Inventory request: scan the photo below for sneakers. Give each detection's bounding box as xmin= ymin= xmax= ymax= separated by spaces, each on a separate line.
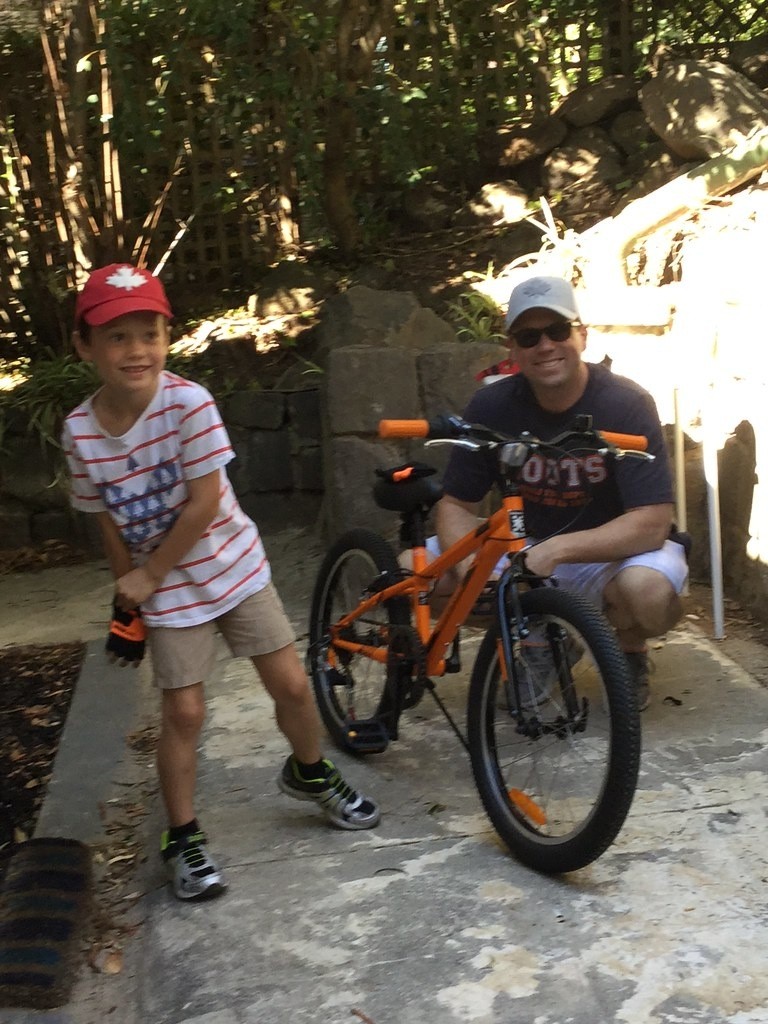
xmin=516 ymin=626 xmax=584 ymax=707
xmin=161 ymin=831 xmax=230 ymax=902
xmin=622 ymin=651 xmax=651 ymax=709
xmin=277 ymin=753 xmax=380 ymax=829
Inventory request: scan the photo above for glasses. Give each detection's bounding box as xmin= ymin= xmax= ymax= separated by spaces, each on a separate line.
xmin=508 ymin=322 xmax=575 ymax=348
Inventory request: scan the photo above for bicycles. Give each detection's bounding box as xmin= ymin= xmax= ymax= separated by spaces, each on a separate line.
xmin=301 ymin=411 xmax=659 ymax=876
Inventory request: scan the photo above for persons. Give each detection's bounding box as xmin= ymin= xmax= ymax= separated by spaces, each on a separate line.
xmin=63 ymin=263 xmax=380 ymax=902
xmin=396 ymin=277 xmax=692 ymax=712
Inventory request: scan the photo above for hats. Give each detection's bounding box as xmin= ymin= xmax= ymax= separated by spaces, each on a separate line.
xmin=508 ymin=277 xmax=577 ymax=330
xmin=75 ymin=264 xmax=174 ymax=325
